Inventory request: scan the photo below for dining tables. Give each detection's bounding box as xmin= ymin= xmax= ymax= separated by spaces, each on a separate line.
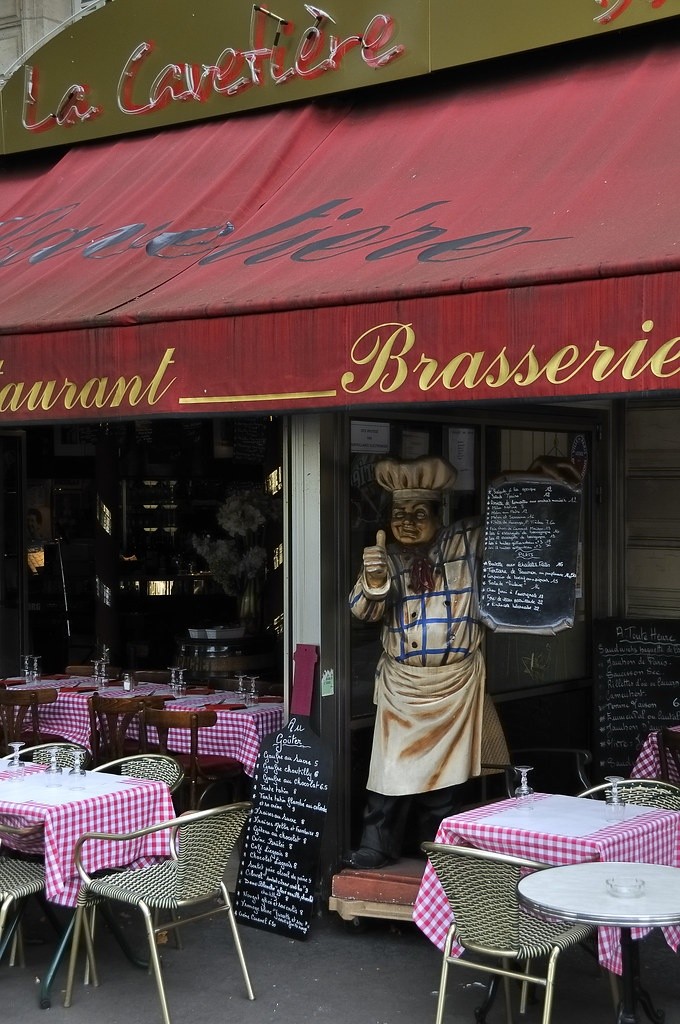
xmin=411 ymin=791 xmax=680 ymax=1024
xmin=0 ymin=674 xmax=284 ymax=810
xmin=0 ymin=760 xmax=177 ymax=1008
xmin=631 ymin=726 xmax=680 ymax=780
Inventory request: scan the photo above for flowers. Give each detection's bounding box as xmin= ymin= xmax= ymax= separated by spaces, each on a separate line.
xmin=192 ymin=482 xmax=277 ymax=598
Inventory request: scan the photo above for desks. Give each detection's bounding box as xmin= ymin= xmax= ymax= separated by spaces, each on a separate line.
xmin=514 ymin=861 xmax=680 ymax=1024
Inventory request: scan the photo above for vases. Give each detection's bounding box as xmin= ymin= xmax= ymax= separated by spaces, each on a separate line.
xmin=240 ymin=577 xmax=264 ymax=640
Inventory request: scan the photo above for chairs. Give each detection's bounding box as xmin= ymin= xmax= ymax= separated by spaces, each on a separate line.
xmin=138 ymin=708 xmax=242 ymax=812
xmin=0 ymin=838 xmax=95 ymax=985
xmin=91 ymin=753 xmax=183 ymax=973
xmin=64 ymin=801 xmax=255 ymax=1024
xmin=422 ymin=840 xmax=620 ymax=1024
xmin=7 ymin=742 xmax=90 ymax=770
xmin=0 ymin=689 xmax=58 ymax=761
xmin=87 ymin=692 xmax=166 ymax=775
xmin=575 ymin=780 xmax=680 ymax=812
xmin=503 ymin=746 xmax=592 ymax=798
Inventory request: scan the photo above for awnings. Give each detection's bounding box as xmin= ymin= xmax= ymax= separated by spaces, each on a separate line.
xmin=0 ymin=27 xmax=680 ymax=421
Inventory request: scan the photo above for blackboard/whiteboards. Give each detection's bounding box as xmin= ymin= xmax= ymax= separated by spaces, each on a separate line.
xmin=595 ymin=616 xmax=680 ymax=776
xmin=233 ymin=715 xmax=334 ymax=942
xmin=475 ymin=473 xmax=582 ymax=635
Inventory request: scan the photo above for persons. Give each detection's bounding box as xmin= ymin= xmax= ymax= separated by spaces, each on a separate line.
xmin=345 ymin=456 xmax=520 ymax=870
xmin=24 ymin=508 xmax=50 ymax=542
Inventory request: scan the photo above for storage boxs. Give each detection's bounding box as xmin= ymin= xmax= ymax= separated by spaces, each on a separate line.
xmin=206 ymin=627 xmax=245 ymax=639
xmin=188 ymin=628 xmax=206 ymax=639
